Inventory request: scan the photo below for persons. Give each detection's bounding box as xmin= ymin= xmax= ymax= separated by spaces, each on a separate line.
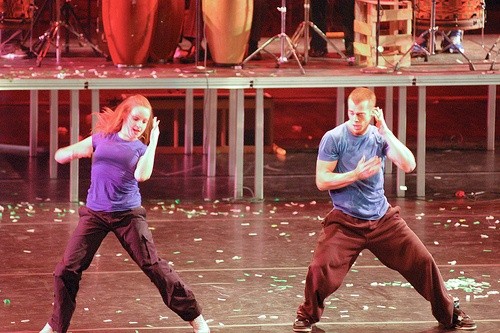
xmin=40 ymin=94 xmax=210 ymax=333
xmin=293 ymin=88 xmax=477 ymax=331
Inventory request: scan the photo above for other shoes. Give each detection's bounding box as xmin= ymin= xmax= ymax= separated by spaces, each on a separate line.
xmin=39 ymin=322 xmax=55 ymax=333
xmin=452 ymin=306 xmax=477 ymax=331
xmin=345 ymin=42 xmax=355 ymax=55
xmin=412 ymin=37 xmax=437 ymax=53
xmin=189 ymin=317 xmax=211 ymax=333
xmin=447 ymin=30 xmax=465 ymax=53
xmin=250 ymin=51 xmax=262 ymax=60
xmin=310 ymin=47 xmax=326 ymax=57
xmin=293 ymin=317 xmax=318 ymax=332
xmin=173 ymin=45 xmax=195 ymax=59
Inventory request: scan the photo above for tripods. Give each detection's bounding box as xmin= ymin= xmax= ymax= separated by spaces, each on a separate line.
xmin=392 ymin=0 xmax=500 ymax=73
xmin=237 ymin=0 xmax=353 ymax=74
xmin=0 ymin=0 xmax=110 ymax=67
